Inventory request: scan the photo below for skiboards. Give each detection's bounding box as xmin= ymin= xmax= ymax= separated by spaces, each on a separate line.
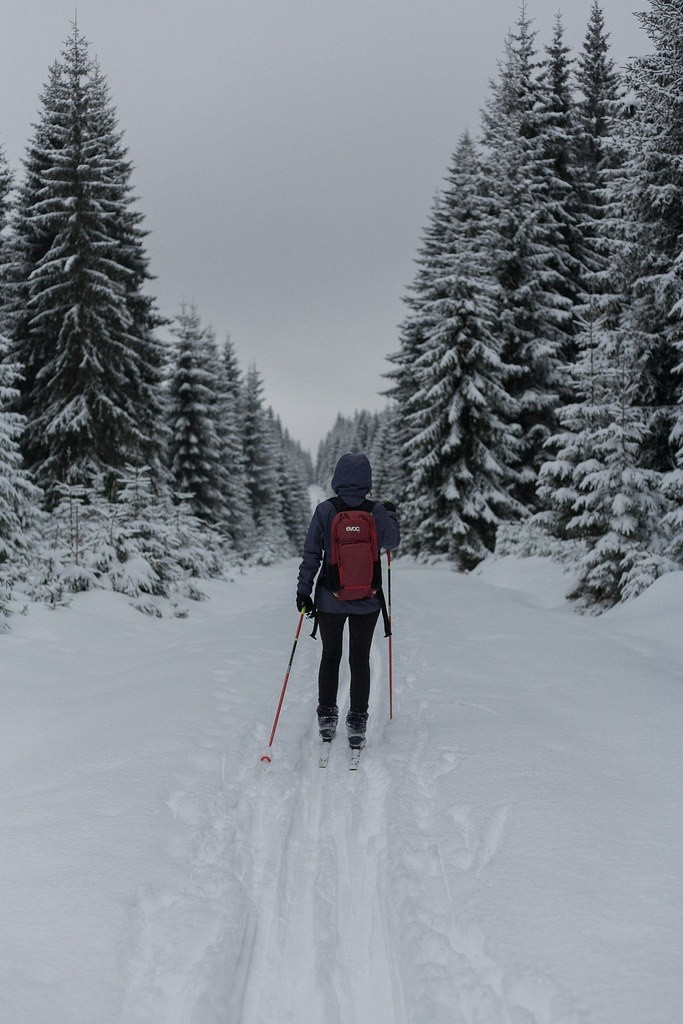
xmin=318 ymin=738 xmax=361 ymax=771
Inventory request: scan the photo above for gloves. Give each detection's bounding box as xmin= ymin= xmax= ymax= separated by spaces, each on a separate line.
xmin=384 ymin=501 xmax=396 ymax=511
xmin=296 ymin=591 xmax=313 ymax=613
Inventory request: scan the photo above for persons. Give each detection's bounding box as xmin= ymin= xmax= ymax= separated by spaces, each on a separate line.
xmin=296 ymin=453 xmax=402 ymax=749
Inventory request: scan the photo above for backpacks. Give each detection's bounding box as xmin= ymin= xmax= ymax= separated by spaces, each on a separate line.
xmin=320 ymin=496 xmax=383 ymax=600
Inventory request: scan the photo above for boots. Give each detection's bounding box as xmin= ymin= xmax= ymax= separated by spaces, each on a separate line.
xmin=346 ymin=711 xmax=368 ymax=744
xmin=316 ymin=705 xmax=338 ymax=739
